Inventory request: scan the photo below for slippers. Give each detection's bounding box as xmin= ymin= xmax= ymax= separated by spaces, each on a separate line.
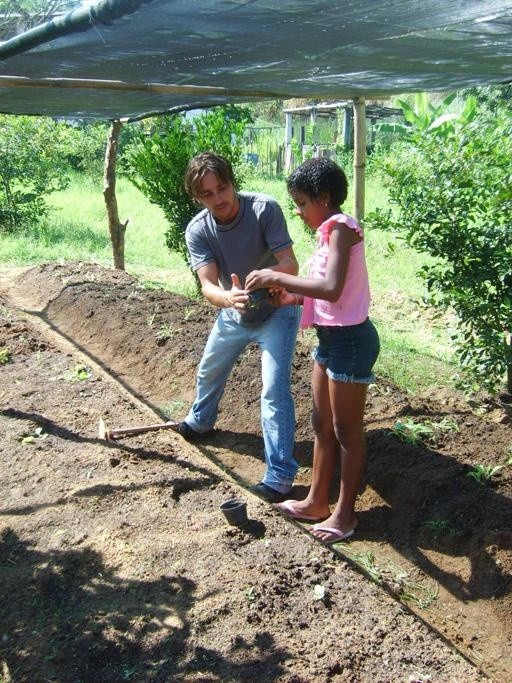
xmin=273 ymin=499 xmax=323 ymax=522
xmin=306 ymin=523 xmax=356 ymax=543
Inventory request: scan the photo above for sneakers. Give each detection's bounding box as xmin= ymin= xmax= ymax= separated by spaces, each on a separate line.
xmin=174 ymin=422 xmax=215 ymax=440
xmin=251 ymin=483 xmax=288 ymax=503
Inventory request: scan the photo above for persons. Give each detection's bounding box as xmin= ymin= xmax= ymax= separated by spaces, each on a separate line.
xmin=241 ymin=155 xmax=383 ymax=549
xmin=179 ymin=150 xmax=299 ymax=498
